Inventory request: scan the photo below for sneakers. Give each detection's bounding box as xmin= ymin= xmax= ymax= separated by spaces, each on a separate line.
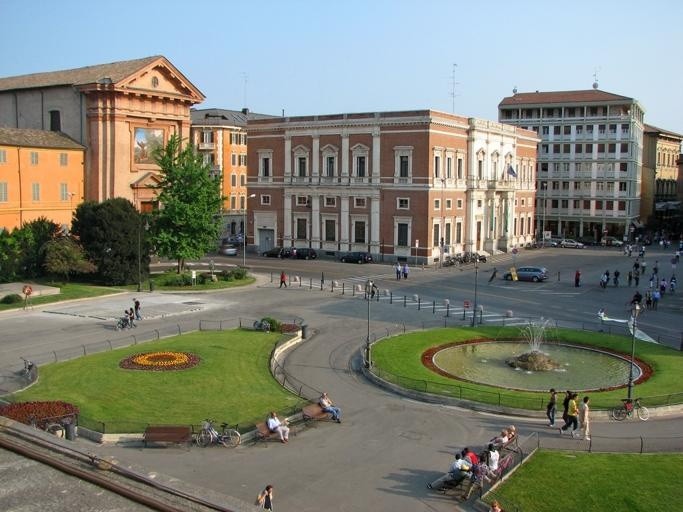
xmin=546 ymin=421 xmax=592 ymax=442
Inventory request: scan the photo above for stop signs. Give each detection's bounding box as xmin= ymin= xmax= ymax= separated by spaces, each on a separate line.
xmin=603 ymin=228 xmax=607 ymax=235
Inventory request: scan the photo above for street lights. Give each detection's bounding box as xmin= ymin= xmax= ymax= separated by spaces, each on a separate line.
xmin=241 ymin=193 xmax=256 ymax=266
xmin=363 ymin=279 xmax=374 ymax=369
xmin=625 ymin=300 xmax=639 ymax=398
xmin=542 ymin=182 xmax=547 ymax=249
xmin=68 ymin=191 xmax=75 ymax=221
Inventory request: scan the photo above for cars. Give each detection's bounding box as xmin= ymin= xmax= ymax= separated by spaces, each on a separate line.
xmin=538 ymin=238 xmax=558 ymax=249
xmin=219 ymin=233 xmax=248 ymax=257
xmin=561 ymin=239 xmax=584 ymax=249
xmin=339 ymin=250 xmax=373 ymax=265
xmin=503 ymin=265 xmax=549 ymax=282
xmin=262 ymin=247 xmax=317 ymax=260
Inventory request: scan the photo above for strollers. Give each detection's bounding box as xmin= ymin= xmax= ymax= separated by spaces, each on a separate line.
xmin=113 ymin=317 xmax=130 ymax=330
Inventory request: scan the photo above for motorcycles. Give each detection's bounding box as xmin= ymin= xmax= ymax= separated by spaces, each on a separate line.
xmin=18 ymin=357 xmax=34 ymax=389
xmin=443 ymin=250 xmax=488 ymax=267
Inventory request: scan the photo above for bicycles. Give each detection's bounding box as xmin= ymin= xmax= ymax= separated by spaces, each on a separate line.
xmin=611 ymin=397 xmax=650 ymax=422
xmin=196 ymin=416 xmax=243 ymax=449
xmin=669 ymin=279 xmax=678 ymax=295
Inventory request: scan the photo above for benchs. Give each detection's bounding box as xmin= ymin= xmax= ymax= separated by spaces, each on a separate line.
xmin=437 ymin=433 xmax=522 ymax=500
xmin=253 ymin=415 xmax=292 ymax=447
xmin=300 ymin=401 xmax=333 ymax=428
xmin=141 ymin=422 xmax=193 ymax=448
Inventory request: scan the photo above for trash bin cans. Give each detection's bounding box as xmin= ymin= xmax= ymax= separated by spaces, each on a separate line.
xmin=49 ymin=418 xmax=75 ymax=441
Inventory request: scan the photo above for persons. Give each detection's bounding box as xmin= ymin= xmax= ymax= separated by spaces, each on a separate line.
xmin=369 ymin=280 xmax=376 ymax=298
xmin=132 ymin=297 xmax=141 ymax=320
xmin=401 ymin=263 xmax=410 ymax=279
xmin=265 ymin=410 xmax=289 ymax=443
xmin=123 ymin=309 xmax=130 ymax=318
xmin=277 ymin=270 xmax=286 ymax=289
xmin=395 ymin=261 xmax=401 ymax=280
xmin=254 ymin=484 xmax=274 ymax=512
xmin=559 ymin=394 xmax=578 ymax=434
xmin=127 ymin=307 xmax=135 ymax=330
xmin=569 ymin=396 xmax=590 ymax=440
xmin=567 ymin=226 xmax=682 ymax=318
xmin=291 ymin=248 xmax=297 ymax=257
xmin=319 ymin=387 xmax=341 ymax=423
xmin=545 ymin=388 xmax=557 ymax=427
xmin=423 ymin=423 xmax=516 ymax=501
xmin=562 ymin=390 xmax=570 ymax=423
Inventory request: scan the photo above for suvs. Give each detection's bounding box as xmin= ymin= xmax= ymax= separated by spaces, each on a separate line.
xmin=600 ymin=236 xmax=624 ymax=247
